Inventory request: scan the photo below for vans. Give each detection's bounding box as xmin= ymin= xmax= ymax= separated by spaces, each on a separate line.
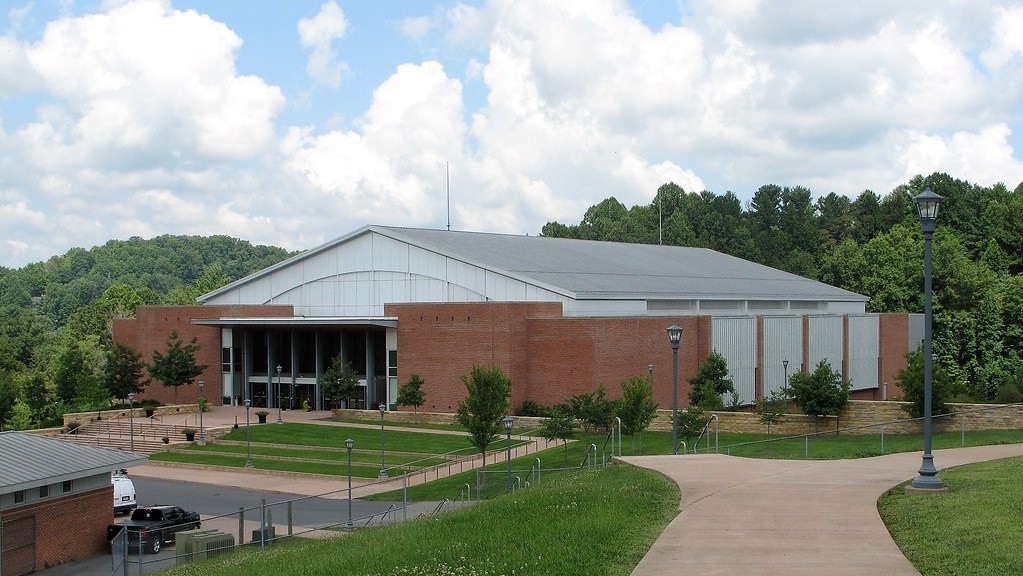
xmin=112 ymin=475 xmax=137 ymax=515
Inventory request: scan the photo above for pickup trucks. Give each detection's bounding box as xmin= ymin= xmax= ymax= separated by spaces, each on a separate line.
xmin=105 ymin=504 xmax=201 ymax=554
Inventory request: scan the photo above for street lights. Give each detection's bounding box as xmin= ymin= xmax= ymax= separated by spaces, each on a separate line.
xmin=276 ymin=365 xmax=282 ymax=421
xmin=648 ymin=363 xmax=654 ymax=410
xmin=198 ymin=381 xmax=206 ymax=440
xmin=783 ymin=358 xmax=788 ymax=408
xmin=129 ymin=392 xmax=135 ymax=452
xmin=378 ymin=402 xmax=388 ymax=475
xmin=244 ymin=398 xmax=253 ymax=466
xmin=666 ymin=321 xmax=683 ymax=453
xmin=914 ymin=185 xmax=942 ymax=490
xmin=932 ymin=354 xmax=937 ymax=383
xmin=502 ymin=415 xmax=514 ymax=493
xmin=345 ymin=438 xmax=354 ymax=529
